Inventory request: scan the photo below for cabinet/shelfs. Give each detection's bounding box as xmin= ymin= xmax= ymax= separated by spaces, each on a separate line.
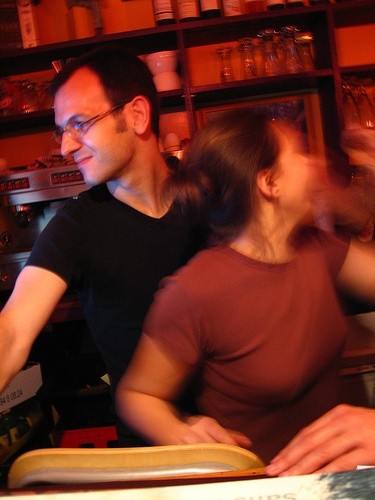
xmin=0 ymin=0 xmax=375 ymax=376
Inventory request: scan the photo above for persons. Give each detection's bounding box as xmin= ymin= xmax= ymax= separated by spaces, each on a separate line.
xmin=114 ymin=105 xmax=375 ymax=478
xmin=0 ymin=50 xmax=222 ymax=449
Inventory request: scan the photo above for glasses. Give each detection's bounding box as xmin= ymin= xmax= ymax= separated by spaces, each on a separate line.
xmin=50 ymin=102 xmax=130 ymax=143
